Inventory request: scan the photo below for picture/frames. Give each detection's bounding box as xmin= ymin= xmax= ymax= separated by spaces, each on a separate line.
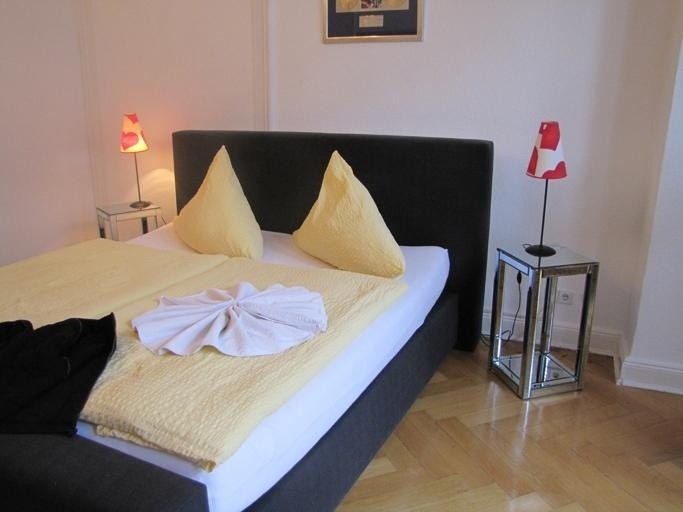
xmin=320 ymin=0 xmax=424 ymax=43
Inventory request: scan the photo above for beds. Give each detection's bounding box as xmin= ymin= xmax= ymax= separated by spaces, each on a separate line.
xmin=0 ymin=129 xmax=494 ymax=512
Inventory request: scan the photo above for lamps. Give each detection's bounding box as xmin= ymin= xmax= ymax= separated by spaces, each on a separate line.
xmin=526 ymin=120 xmax=568 ymax=257
xmin=120 ymin=112 xmax=150 ymax=208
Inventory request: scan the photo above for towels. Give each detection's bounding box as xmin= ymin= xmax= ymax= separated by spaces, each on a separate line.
xmin=131 ymin=281 xmax=329 ymax=358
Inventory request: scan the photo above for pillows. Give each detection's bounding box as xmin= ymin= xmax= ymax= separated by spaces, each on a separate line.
xmin=172 ymin=144 xmax=407 ymax=278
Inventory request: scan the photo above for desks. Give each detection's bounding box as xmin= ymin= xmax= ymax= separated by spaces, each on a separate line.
xmin=489 ymin=244 xmax=602 ymax=401
xmin=95 ymin=202 xmax=161 ymax=241
